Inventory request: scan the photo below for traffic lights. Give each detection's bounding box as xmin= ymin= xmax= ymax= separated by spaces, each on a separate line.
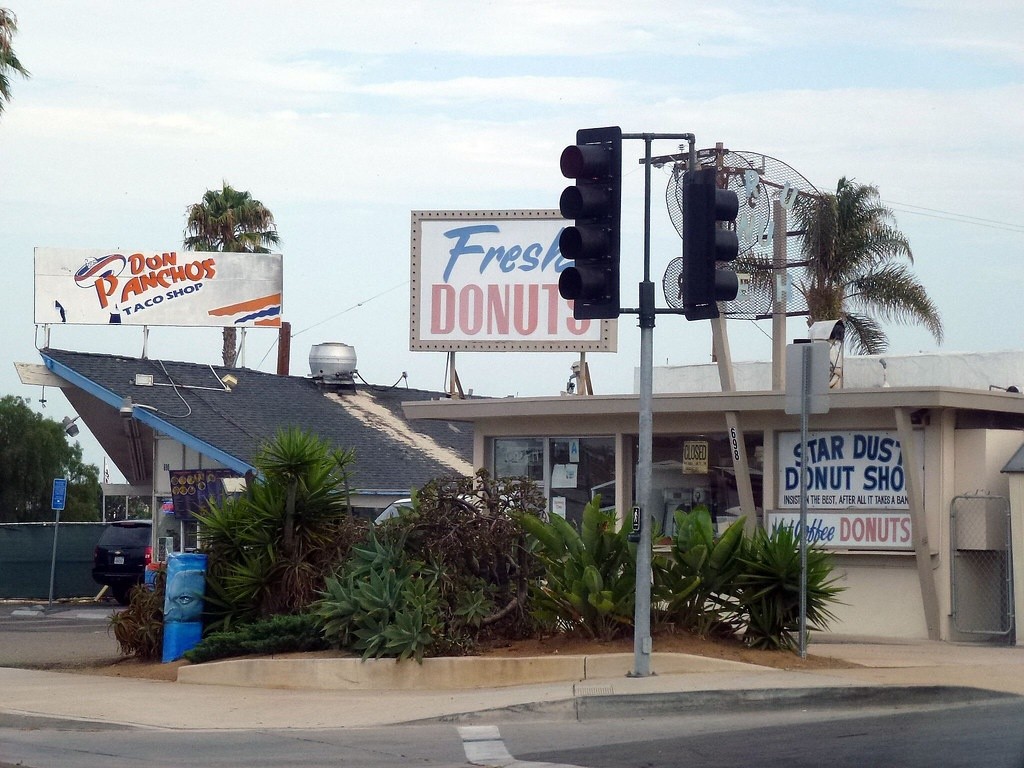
xmin=560 ymin=127 xmax=621 ymax=320
xmin=682 ymin=167 xmax=740 ymax=320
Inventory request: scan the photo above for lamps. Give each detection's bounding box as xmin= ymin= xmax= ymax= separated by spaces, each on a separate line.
xmin=221 ymin=374 xmax=238 ymax=393
xmin=62 ymin=415 xmax=81 ymax=437
xmin=135 ymin=374 xmax=153 ymax=387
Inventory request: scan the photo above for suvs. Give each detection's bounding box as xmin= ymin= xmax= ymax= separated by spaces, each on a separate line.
xmin=374 ymin=499 xmax=508 ymax=522
xmin=91 ymin=500 xmax=176 ymax=607
